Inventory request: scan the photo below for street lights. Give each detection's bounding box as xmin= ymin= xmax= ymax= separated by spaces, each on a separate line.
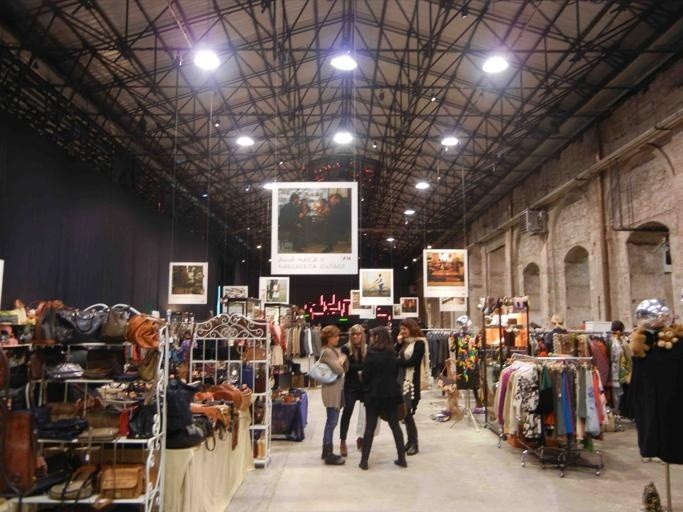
xmin=27 ymin=405 xmax=162 ymax=441
xmin=307 ymin=350 xmax=338 ymax=384
xmin=166 ymin=366 xmax=195 ymax=432
xmin=102 ymin=468 xmax=142 ymax=499
xmin=125 ymin=314 xmax=164 ymax=348
xmin=381 ymin=399 xmax=408 ymax=421
xmin=50 ymin=471 xmax=93 ymax=504
xmin=101 ymin=310 xmax=127 ymax=342
xmin=32 ymin=300 xmax=63 ymax=342
xmin=26 ymin=348 xmax=159 ymax=382
xmin=55 ymin=307 xmax=106 ymax=342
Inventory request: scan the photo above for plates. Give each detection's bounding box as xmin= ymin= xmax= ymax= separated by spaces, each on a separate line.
xmin=0 ymin=304 xmax=168 ymax=512
xmin=481 ymin=296 xmax=529 ymax=427
xmin=187 ymin=313 xmax=274 ymax=469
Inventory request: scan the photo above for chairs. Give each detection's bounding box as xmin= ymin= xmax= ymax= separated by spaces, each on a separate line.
xmin=395 ymin=459 xmax=407 ymax=467
xmin=359 ymin=462 xmax=367 ymax=470
xmin=407 ymin=445 xmax=417 ymax=455
xmin=405 ymin=442 xmax=409 ymax=451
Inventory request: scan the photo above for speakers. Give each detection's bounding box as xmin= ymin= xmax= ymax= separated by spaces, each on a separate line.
xmin=324 ymin=444 xmax=345 ymax=465
xmin=340 ymin=440 xmax=346 ymax=456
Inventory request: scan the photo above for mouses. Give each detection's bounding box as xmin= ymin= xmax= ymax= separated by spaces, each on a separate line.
xmin=542 ymin=327 xmax=629 ymax=340
xmin=425 ymin=326 xmax=455 ymax=335
xmin=646 ymin=310 xmax=680 ymax=330
xmin=507 ymin=355 xmax=599 ymax=384
xmin=509 ymin=352 xmax=542 ymax=364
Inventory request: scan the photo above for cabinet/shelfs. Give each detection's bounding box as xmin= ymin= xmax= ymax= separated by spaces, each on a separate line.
xmin=481 ymin=296 xmax=529 ymax=427
xmin=0 ymin=304 xmax=168 ymax=512
xmin=187 ymin=313 xmax=274 ymax=469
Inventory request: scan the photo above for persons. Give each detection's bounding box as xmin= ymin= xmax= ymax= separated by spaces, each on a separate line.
xmin=377 ymin=275 xmax=384 ymax=292
xmin=544 ymin=313 xmax=567 ymax=353
xmin=339 ymin=325 xmax=371 ymax=456
xmin=359 ymin=328 xmax=408 ymax=470
xmin=278 ymin=194 xmax=346 ymax=251
xmin=172 ymin=330 xmax=198 ymax=364
xmin=317 ymin=325 xmax=344 ymax=465
xmin=271 ymin=280 xmax=280 ymax=302
xmin=393 ymin=318 xmax=424 ymax=454
xmin=613 ymin=320 xmax=624 ymax=330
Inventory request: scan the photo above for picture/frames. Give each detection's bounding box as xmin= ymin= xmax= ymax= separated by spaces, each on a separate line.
xmin=164 ymin=403 xmax=255 ymax=512
xmin=272 ymin=388 xmax=308 ymax=442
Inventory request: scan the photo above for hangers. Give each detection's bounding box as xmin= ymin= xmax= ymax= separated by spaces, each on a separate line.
xmin=425 ymin=326 xmax=455 ymax=335
xmin=509 ymin=352 xmax=542 ymax=364
xmin=542 ymin=327 xmax=629 ymax=340
xmin=646 ymin=310 xmax=680 ymax=330
xmin=507 ymin=355 xmax=599 ymax=384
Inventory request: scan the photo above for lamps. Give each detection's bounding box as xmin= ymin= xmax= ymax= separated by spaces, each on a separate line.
xmin=191 ymin=42 xmax=512 ymax=271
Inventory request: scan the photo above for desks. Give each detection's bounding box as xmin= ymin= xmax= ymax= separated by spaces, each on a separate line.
xmin=164 ymin=403 xmax=255 ymax=512
xmin=272 ymin=388 xmax=308 ymax=442
